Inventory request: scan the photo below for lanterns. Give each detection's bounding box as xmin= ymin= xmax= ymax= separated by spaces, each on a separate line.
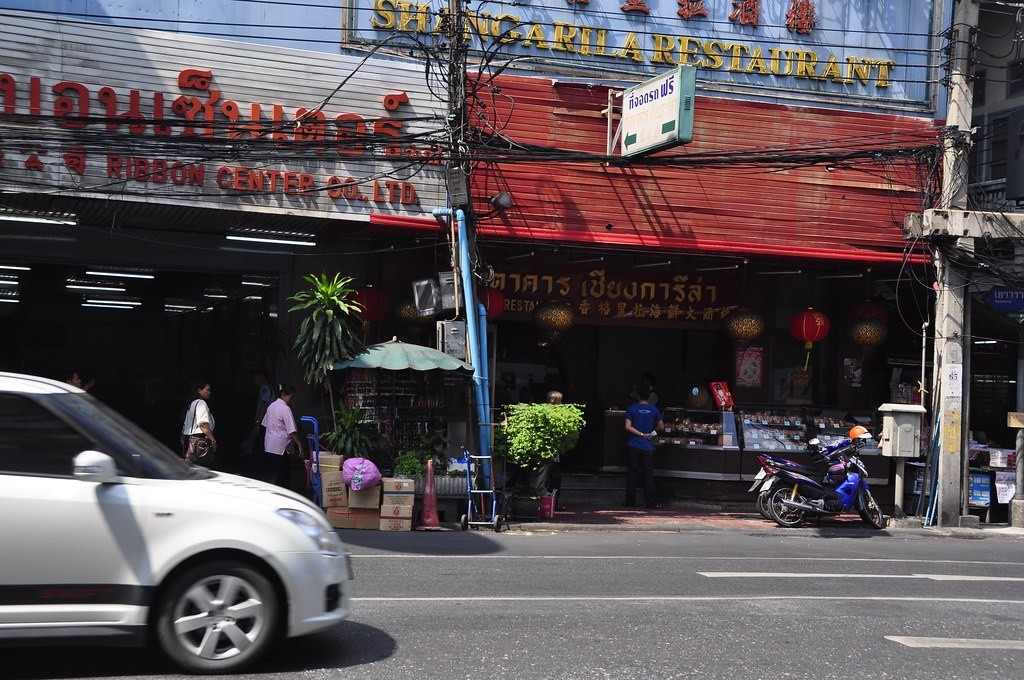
xmin=789 ymin=306 xmax=830 ymax=351
xmin=847 ymin=315 xmax=889 ymax=351
xmin=724 ymin=310 xmax=765 ymax=343
xmin=348 ymin=286 xmax=388 ymax=324
xmin=478 ymin=287 xmax=506 ymax=321
xmin=532 ymin=299 xmax=575 ymax=336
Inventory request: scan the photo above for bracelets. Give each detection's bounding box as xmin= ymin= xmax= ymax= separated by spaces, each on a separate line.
xmin=651 ymin=430 xmax=658 ymax=437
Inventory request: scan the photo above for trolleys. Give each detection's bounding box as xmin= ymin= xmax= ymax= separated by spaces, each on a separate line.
xmin=300 ymin=415 xmax=324 ymax=509
xmin=460 ymin=445 xmax=510 ymax=533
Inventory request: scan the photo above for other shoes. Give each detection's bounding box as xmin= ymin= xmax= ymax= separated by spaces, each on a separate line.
xmin=647 ymin=503 xmax=665 ymax=509
xmin=555 ymin=504 xmax=566 ymax=511
xmin=620 ymin=501 xmax=640 ymax=507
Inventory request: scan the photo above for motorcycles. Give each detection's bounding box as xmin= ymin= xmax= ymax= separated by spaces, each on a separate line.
xmin=747 ymin=432 xmax=883 ymax=530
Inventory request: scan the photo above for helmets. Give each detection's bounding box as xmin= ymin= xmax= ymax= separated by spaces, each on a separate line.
xmin=850 ymin=426 xmax=872 ymax=440
xmin=827 ymin=463 xmax=847 ymax=483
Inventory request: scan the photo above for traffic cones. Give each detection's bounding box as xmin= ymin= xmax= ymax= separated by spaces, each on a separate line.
xmin=415 ymin=460 xmax=453 ymax=532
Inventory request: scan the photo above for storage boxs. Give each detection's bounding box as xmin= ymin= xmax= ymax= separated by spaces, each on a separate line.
xmin=541 ymin=494 xmax=556 ymax=518
xmin=990 ymin=450 xmax=1016 ymax=467
xmin=312 ymin=450 xmax=416 ymax=532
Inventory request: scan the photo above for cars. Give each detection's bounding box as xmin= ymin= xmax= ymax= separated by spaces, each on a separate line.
xmin=0 ymin=372 xmax=355 ymax=673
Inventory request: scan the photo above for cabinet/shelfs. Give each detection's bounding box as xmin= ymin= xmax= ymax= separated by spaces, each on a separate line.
xmin=655 ymin=406 xmax=877 ymax=450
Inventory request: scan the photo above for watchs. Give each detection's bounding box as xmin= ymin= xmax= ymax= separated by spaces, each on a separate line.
xmin=211 ymin=440 xmax=216 ymax=444
xmin=641 ymin=432 xmax=644 ymax=437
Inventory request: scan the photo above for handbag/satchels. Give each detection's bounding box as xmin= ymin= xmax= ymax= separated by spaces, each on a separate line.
xmin=540 ymin=496 xmax=554 ymax=518
xmin=186 ymin=437 xmax=215 ymax=463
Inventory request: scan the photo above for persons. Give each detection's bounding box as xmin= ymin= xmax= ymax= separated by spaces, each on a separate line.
xmin=62 ymin=370 xmax=100 ymax=400
xmin=618 ymin=384 xmax=665 ymax=507
xmin=181 ymin=379 xmax=219 ymax=460
xmin=261 ymin=385 xmax=306 ymax=474
xmin=542 ymin=391 xmax=566 ymax=511
xmin=249 ymin=364 xmax=276 ymax=462
xmin=639 ymin=372 xmax=664 ymax=407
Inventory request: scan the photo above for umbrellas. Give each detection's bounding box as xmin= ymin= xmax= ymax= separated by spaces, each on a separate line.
xmin=331 ymin=335 xmax=475 ymax=472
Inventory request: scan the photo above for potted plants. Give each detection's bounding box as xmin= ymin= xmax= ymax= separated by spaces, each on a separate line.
xmin=392 ymin=436 xmax=476 ymax=496
xmin=492 ymin=402 xmax=587 ymax=519
